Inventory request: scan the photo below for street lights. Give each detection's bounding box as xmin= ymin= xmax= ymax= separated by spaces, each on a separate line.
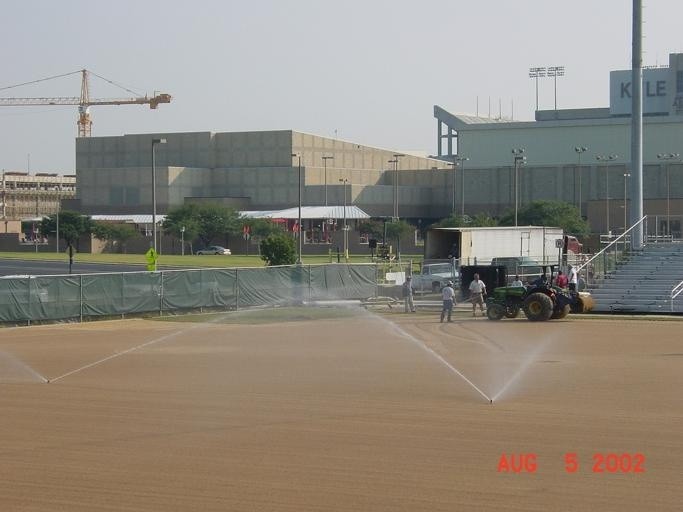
xmin=548 ymin=64 xmax=564 ymax=111
xmin=445 ymin=163 xmax=460 ymax=215
xmin=622 ymin=173 xmax=631 ymax=251
xmin=337 ymin=178 xmax=350 ymax=257
xmin=52 ymin=185 xmax=60 ymax=254
xmin=394 ymin=154 xmax=404 ymax=222
xmin=321 ymin=155 xmax=333 ymax=206
xmin=148 ymin=136 xmax=169 ymax=272
xmin=572 ymin=146 xmax=589 ymax=224
xmin=595 ymin=154 xmax=619 ymax=240
xmin=292 ymin=151 xmax=303 ymax=267
xmin=510 ymin=148 xmax=526 ymax=228
xmin=514 ymin=156 xmax=527 ymax=208
xmin=456 ymin=157 xmax=468 ymax=216
xmin=529 ymin=67 xmax=548 ymax=111
xmin=657 ymin=151 xmax=680 ymax=237
xmin=388 ymin=159 xmax=399 ymax=218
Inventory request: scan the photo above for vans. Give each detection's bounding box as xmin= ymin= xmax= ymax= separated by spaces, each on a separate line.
xmin=489 ymin=255 xmax=555 ymax=289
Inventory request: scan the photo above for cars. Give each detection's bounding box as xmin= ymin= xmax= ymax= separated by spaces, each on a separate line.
xmin=195 ymin=245 xmax=231 ymax=255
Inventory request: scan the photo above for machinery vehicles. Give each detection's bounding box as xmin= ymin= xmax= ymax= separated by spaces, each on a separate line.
xmin=480 ymin=261 xmax=598 ymax=323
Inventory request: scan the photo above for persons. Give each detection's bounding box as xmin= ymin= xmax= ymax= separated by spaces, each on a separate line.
xmin=511 ymin=274 xmax=523 ymax=287
xmin=555 ymin=270 xmax=568 ymax=288
xmin=440 ymin=280 xmax=457 ymax=323
xmin=468 ymin=272 xmax=488 ymax=317
xmin=566 ymin=263 xmax=578 ymax=313
xmin=401 ymin=276 xmax=416 ymax=313
xmin=529 ymin=275 xmax=546 ymax=286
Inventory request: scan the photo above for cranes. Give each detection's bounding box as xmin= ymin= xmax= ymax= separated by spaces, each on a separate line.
xmin=0 ymin=68 xmax=171 ymax=138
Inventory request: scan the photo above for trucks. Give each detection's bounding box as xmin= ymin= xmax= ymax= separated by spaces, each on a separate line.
xmin=399 ymin=261 xmax=459 ymax=294
xmin=419 ymin=221 xmax=588 ymax=281
